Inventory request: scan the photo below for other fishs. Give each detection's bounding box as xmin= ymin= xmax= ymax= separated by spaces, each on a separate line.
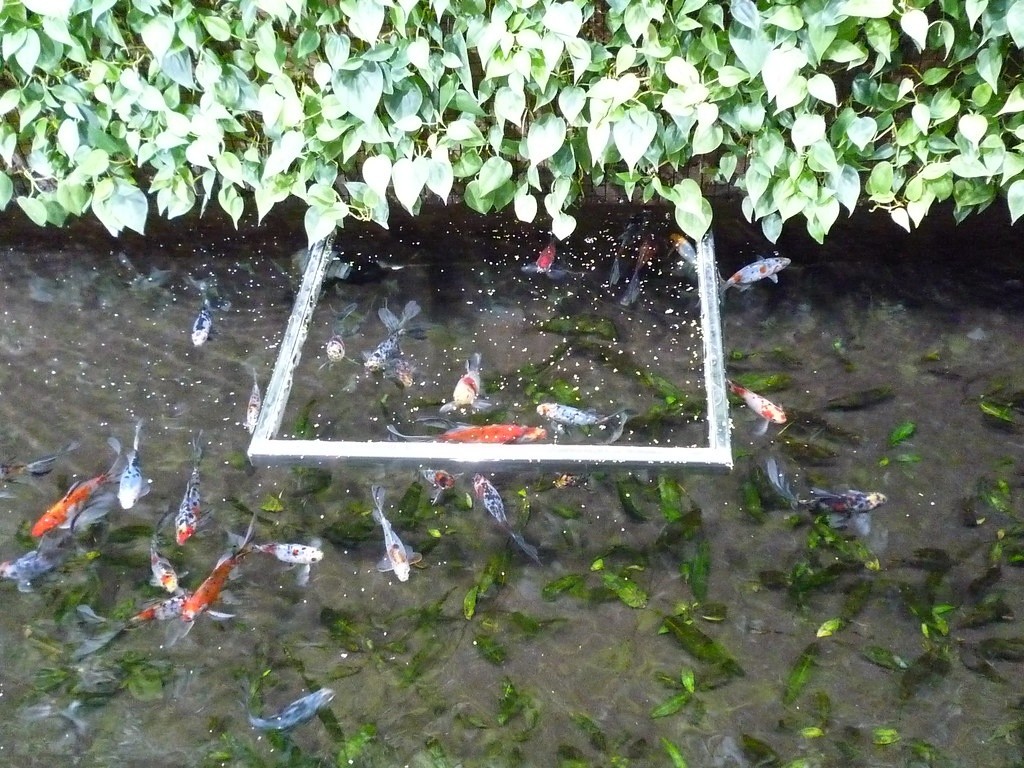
xmin=0 ymin=236 xmax=896 ymax=766
